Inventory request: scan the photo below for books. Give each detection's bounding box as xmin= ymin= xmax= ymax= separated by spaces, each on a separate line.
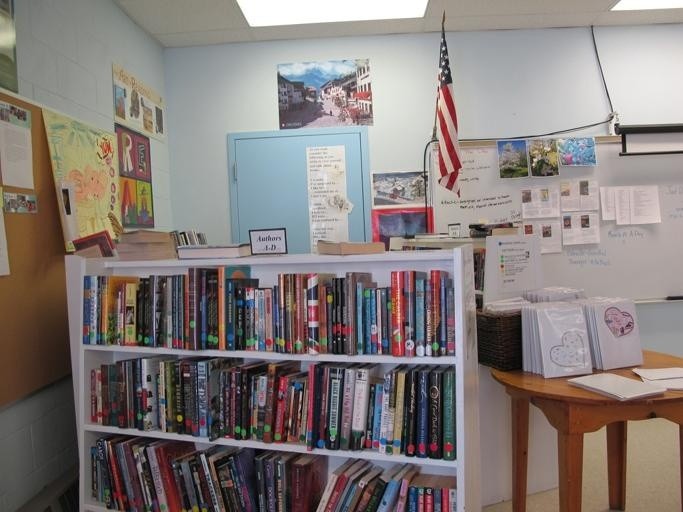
xmin=176 ymin=244 xmax=251 ymax=259
xmin=169 ymin=229 xmax=207 ymax=245
xmin=72 ymin=244 xmax=102 ymax=257
xmin=475 ymin=285 xmax=645 ymax=381
xmin=71 ymin=230 xmax=117 ymax=257
xmin=567 ymin=371 xmax=666 ymax=405
xmin=90 ymin=357 xmax=455 ymax=460
xmin=83 ymin=265 xmax=454 ymax=357
xmin=115 ymin=230 xmax=175 ymax=261
xmin=90 ymin=436 xmax=457 ymax=511
xmin=316 ymin=240 xmax=386 ymax=256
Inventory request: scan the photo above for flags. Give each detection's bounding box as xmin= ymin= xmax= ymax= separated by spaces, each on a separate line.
xmin=435 ymin=21 xmax=462 ymax=198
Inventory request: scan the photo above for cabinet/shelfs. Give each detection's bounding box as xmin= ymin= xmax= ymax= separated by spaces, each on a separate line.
xmin=65 ymin=244 xmax=483 ymax=512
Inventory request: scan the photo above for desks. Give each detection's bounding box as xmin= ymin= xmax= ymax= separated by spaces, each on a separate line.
xmin=490 ymin=349 xmax=683 ymax=512
